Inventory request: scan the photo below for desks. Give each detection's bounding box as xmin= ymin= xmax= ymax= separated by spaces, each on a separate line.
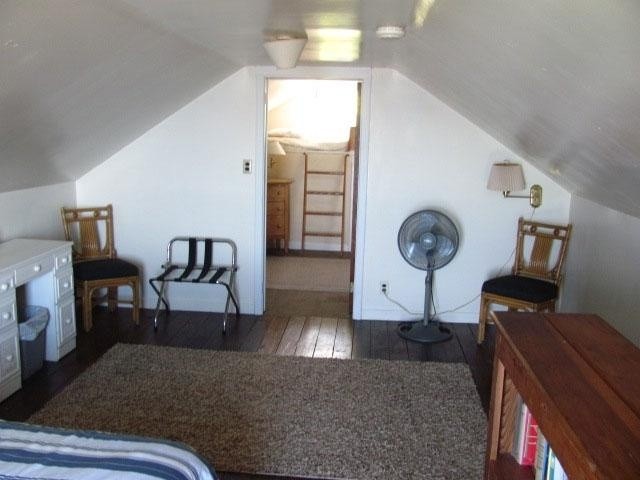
xmin=1 ymin=237 xmax=77 ymax=404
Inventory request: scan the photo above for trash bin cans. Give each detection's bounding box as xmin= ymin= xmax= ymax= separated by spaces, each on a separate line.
xmin=18 ymin=305 xmax=50 ymax=381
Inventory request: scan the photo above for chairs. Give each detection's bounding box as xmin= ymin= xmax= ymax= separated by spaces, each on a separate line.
xmin=61 ymin=204 xmax=141 ymax=332
xmin=149 ymin=237 xmax=240 ymax=335
xmin=477 ymin=217 xmax=572 ymax=343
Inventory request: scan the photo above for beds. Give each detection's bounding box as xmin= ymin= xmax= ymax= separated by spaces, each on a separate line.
xmin=0 ymin=417 xmax=219 ymax=479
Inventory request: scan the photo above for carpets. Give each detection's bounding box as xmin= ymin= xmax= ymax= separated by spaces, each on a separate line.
xmin=19 ymin=342 xmax=490 ymax=479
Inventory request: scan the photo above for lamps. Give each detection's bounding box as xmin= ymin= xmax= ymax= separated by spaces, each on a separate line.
xmin=485 ymin=160 xmax=542 ymax=207
xmin=261 ymin=33 xmax=307 ymax=71
xmin=267 ymin=141 xmax=286 ymax=179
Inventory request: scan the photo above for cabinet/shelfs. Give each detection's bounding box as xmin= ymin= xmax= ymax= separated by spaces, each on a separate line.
xmin=481 ymin=310 xmax=638 ymax=479
xmin=267 ymin=179 xmax=292 ymax=255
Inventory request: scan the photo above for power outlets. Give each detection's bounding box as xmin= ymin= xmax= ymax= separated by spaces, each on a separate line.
xmin=380 ymin=282 xmax=390 ymax=297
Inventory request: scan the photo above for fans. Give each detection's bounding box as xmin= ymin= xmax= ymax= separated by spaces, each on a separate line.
xmin=398 ymin=209 xmax=459 ymax=344
